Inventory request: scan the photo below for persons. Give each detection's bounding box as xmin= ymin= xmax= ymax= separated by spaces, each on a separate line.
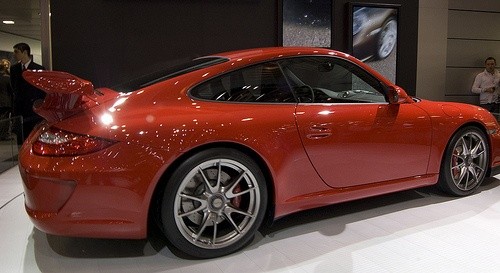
xmin=0 ymin=42 xmax=46 ymax=151
xmin=472 ymin=57 xmax=500 ymax=113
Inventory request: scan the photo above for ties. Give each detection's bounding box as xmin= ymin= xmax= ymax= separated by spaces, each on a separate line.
xmin=23 ymin=64 xmax=26 ymax=70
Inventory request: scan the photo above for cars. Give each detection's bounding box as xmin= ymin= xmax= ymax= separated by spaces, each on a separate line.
xmin=18 ymin=45 xmax=500 ymax=259
